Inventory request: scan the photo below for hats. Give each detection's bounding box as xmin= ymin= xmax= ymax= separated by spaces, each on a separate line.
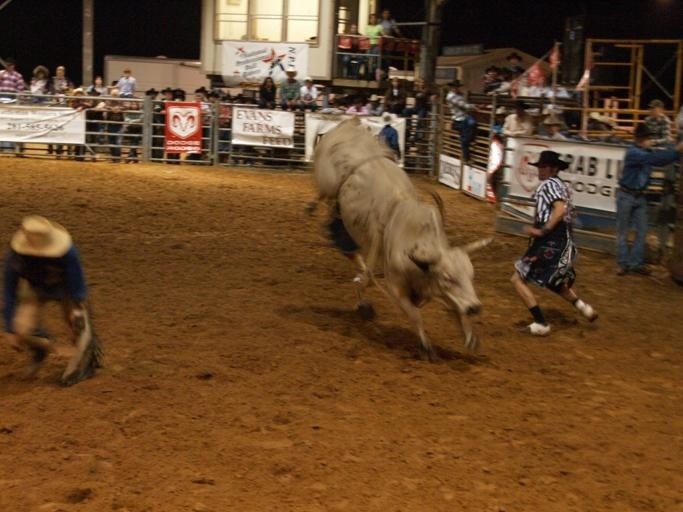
xmin=632 ymin=123 xmax=657 ymax=137
xmin=145 ymin=86 xmax=254 ymax=105
xmin=285 ymin=66 xmax=297 ymax=72
xmin=484 ymin=66 xmax=502 ymax=74
xmin=528 ymin=151 xmax=571 ymax=170
xmin=505 ymin=51 xmax=522 ymax=60
xmin=10 ymin=216 xmax=73 ymax=259
xmin=34 ymin=66 xmax=49 ymax=77
xmin=382 ymin=112 xmax=394 ymax=125
xmin=447 ymin=80 xmax=464 ymax=88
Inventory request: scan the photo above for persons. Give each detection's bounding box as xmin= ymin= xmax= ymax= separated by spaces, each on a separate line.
xmin=362 ymin=13 xmax=386 ymax=81
xmin=443 ymin=50 xmax=681 ymax=239
xmin=376 ymin=7 xmax=400 ymax=40
xmin=340 ymin=22 xmax=366 ymax=79
xmin=1 ymin=59 xmax=435 ymax=172
xmin=1 ymin=212 xmax=96 ymax=385
xmin=611 ymin=122 xmax=681 ymax=276
xmin=510 ymin=149 xmax=597 ymax=337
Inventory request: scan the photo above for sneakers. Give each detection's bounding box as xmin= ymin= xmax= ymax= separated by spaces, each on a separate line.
xmin=581 ymin=303 xmax=598 ymax=321
xmin=630 ymin=266 xmax=651 ymax=274
xmin=149 ymin=156 xmax=290 ymax=168
xmin=527 ymin=322 xmax=550 ymax=335
xmin=16 ymin=154 xmax=21 ymax=158
xmin=20 ymin=154 xmax=24 ymax=158
xmin=47 ymin=152 xmax=139 ymax=163
xmin=470 ymin=143 xmax=475 ymax=146
xmin=463 ymin=160 xmax=473 ymax=165
xmin=615 ymin=265 xmax=627 ymax=275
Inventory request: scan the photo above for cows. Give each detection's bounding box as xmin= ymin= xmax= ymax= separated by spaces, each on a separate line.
xmin=303 ymin=113 xmax=496 ymax=365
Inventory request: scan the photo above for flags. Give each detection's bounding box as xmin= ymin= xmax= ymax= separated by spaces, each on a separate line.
xmin=336 ymin=35 xmax=419 ymax=55
